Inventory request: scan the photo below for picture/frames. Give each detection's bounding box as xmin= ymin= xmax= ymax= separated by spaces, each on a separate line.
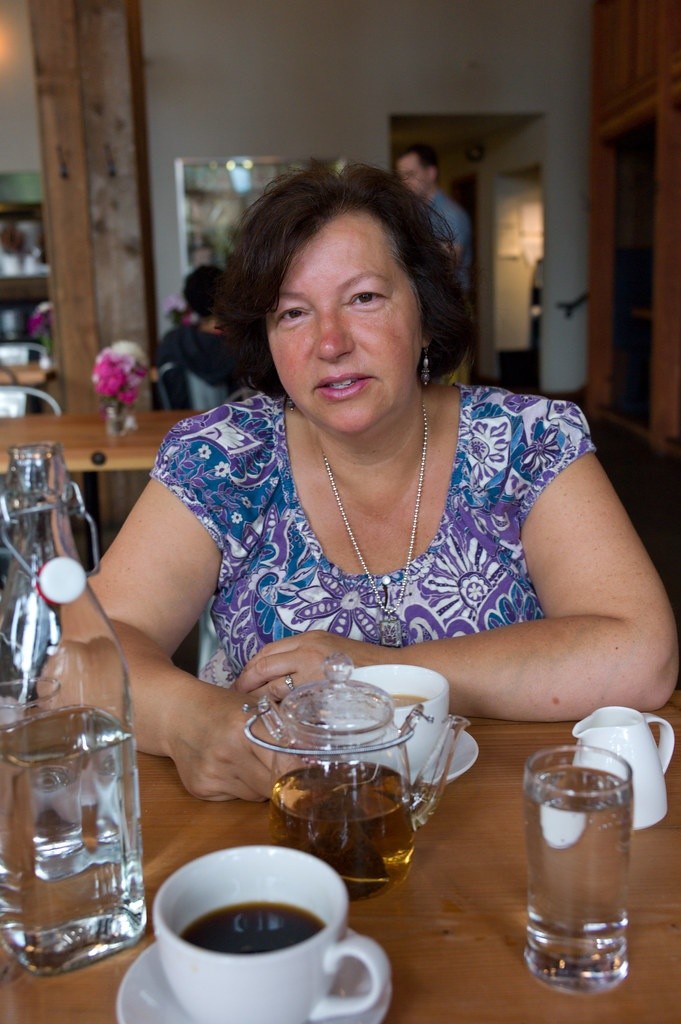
xmin=175 ymin=153 xmax=309 ymax=276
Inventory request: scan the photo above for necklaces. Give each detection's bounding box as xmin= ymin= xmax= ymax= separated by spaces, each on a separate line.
xmin=321 ymin=401 xmax=427 ymax=647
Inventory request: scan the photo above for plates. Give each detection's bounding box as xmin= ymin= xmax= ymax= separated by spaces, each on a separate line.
xmin=414 ymin=727 xmax=479 ymax=784
xmin=115 ymin=922 xmax=392 ymax=1024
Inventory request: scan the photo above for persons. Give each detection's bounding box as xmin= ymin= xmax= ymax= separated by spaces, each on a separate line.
xmin=155 ymin=265 xmax=238 ymax=410
xmin=396 ymin=143 xmax=469 ymax=384
xmin=38 ymin=157 xmax=679 ymax=802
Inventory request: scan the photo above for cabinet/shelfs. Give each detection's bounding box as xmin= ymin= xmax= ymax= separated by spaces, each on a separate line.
xmin=591 ymin=1 xmax=681 ymax=454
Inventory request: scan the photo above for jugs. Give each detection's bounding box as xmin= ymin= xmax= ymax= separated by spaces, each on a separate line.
xmin=243 ymin=653 xmax=470 ymax=900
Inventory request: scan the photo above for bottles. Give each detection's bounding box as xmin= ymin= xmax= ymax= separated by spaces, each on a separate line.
xmin=0 ymin=443 xmax=148 ymax=979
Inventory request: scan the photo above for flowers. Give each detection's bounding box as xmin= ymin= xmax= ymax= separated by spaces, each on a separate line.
xmin=30 ymin=299 xmax=57 ymax=359
xmin=93 ymin=339 xmax=149 ymax=414
xmin=165 ymin=292 xmax=192 ymax=327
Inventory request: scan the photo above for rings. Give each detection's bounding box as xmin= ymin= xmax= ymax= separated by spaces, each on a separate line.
xmin=286 ymin=675 xmax=294 ymax=691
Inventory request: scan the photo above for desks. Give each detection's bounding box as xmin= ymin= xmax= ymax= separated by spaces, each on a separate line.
xmin=1 ymin=687 xmax=680 ymax=1024
xmin=0 ymin=408 xmax=210 ymax=569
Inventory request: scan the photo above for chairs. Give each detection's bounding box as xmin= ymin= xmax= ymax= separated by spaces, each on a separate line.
xmin=0 ymin=385 xmax=100 ymax=587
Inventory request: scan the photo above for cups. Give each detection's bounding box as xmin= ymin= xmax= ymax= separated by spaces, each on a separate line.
xmin=348 ymin=665 xmax=449 ymax=773
xmin=570 ymin=705 xmax=676 ymax=829
xmin=153 ymin=846 xmax=388 ymax=1024
xmin=522 ymin=746 xmax=636 ymax=994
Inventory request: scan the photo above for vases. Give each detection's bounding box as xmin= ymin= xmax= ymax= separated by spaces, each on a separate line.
xmin=105 ymin=404 xmax=125 ymax=437
